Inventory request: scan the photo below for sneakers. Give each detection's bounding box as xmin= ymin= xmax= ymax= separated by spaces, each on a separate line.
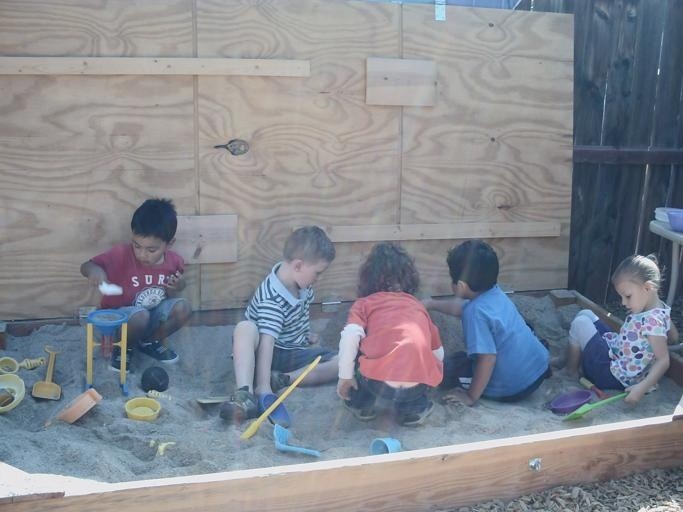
xmin=271 ymin=367 xmax=291 ymax=394
xmin=343 ymin=387 xmax=376 ymax=421
xmin=400 ymin=400 xmax=435 ymax=426
xmin=108 ymin=342 xmax=134 ymax=375
xmin=137 ymin=337 xmax=180 ymax=364
xmin=220 ymin=386 xmax=259 ymax=421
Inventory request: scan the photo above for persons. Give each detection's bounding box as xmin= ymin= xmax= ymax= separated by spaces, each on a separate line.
xmin=215 ymin=225 xmax=339 ymax=421
xmin=420 ymin=237 xmax=550 ymax=408
xmin=334 ymin=240 xmax=444 ymax=426
xmin=542 ymin=254 xmax=680 ymax=408
xmin=80 ymin=198 xmax=194 ymax=373
